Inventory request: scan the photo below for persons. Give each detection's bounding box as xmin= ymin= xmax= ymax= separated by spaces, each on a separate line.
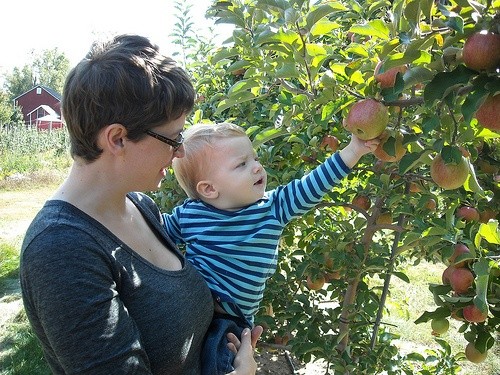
xmin=19 ymin=34 xmax=263 ymax=375
xmin=160 ymin=122 xmax=380 ymax=375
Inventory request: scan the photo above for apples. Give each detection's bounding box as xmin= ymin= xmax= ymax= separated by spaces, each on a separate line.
xmin=261 ymin=29 xmax=500 ymax=364
xmin=198 ymin=67 xmax=245 ymax=100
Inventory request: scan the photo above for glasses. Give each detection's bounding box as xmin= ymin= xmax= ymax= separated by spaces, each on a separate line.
xmin=142 ymin=129 xmax=184 ymax=152
